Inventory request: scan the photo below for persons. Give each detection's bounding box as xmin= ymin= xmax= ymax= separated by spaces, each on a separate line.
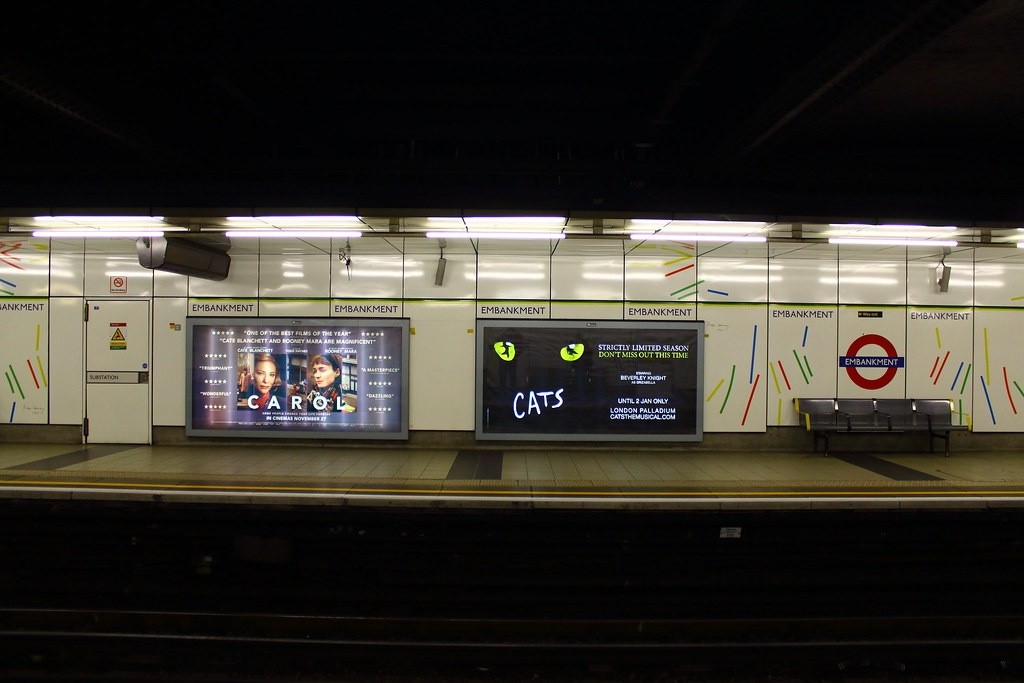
xmin=252 ymin=353 xmax=286 ymax=412
xmin=238 ymin=369 xmax=253 ymax=398
xmin=291 ymin=384 xmax=298 ymax=395
xmin=307 ymin=353 xmax=343 ymax=413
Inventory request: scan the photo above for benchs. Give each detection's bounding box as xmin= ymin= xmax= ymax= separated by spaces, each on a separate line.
xmin=791 ymin=399 xmax=969 ymax=458
